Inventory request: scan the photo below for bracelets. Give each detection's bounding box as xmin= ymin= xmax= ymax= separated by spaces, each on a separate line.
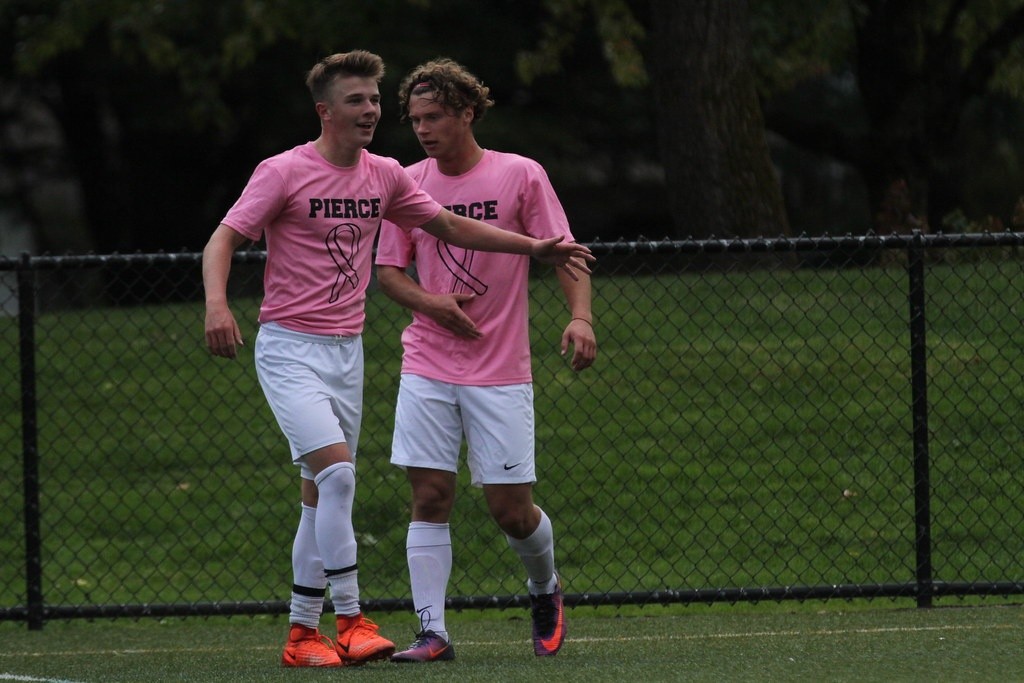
xmin=571 ymin=316 xmax=595 ymax=328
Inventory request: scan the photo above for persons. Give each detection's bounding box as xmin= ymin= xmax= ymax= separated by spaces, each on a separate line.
xmin=369 ymin=59 xmax=597 ymax=663
xmin=198 ymin=50 xmax=599 ymax=669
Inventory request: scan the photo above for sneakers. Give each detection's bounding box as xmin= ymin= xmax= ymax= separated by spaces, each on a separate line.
xmin=335 ymin=611 xmax=395 ymax=666
xmin=391 ymin=628 xmax=455 ymax=663
xmin=527 ymin=571 xmax=568 ymax=656
xmin=279 ymin=623 xmax=343 ymax=667
